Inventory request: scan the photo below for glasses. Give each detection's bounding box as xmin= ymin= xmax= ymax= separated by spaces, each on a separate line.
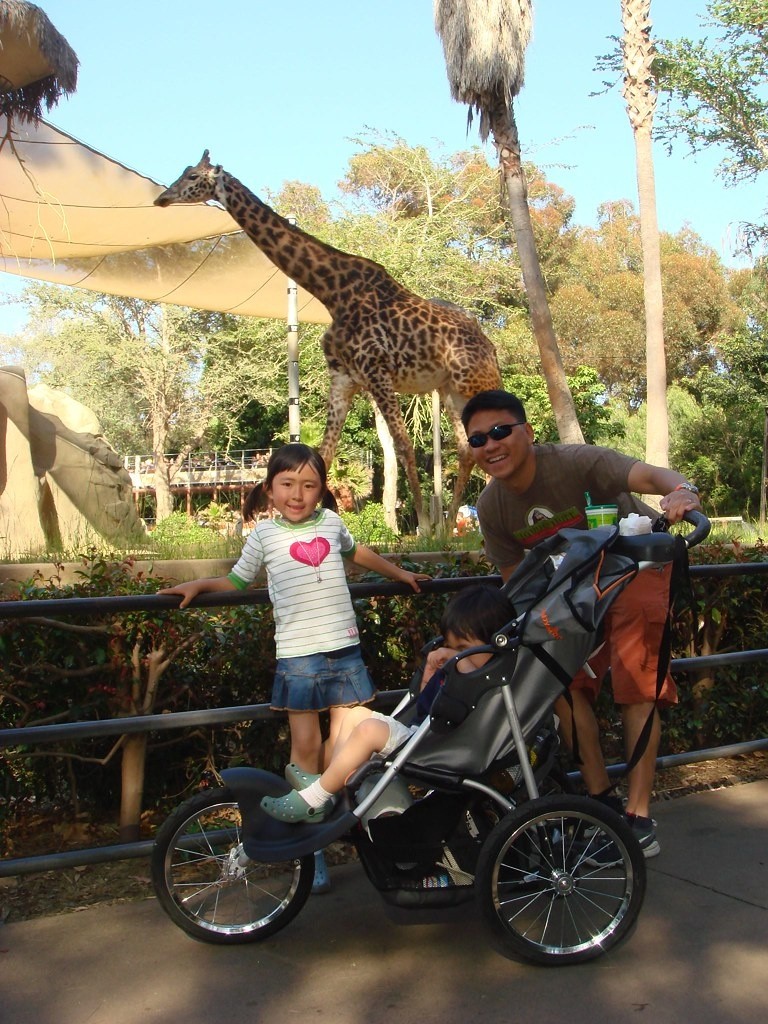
xmin=467 ymin=422 xmax=525 ymax=448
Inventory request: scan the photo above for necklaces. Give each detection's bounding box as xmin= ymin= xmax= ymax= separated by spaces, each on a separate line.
xmin=280 ymin=516 xmax=322 ymax=583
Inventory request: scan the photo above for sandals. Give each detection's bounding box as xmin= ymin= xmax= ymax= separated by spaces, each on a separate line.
xmin=260 ymin=789 xmax=328 ymax=823
xmin=285 ymin=763 xmax=334 ymax=817
xmin=311 ymin=850 xmax=331 ymax=894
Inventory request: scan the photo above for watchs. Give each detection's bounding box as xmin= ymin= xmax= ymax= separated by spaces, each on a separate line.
xmin=675 ymin=482 xmax=698 ymax=494
xmin=460 ymin=390 xmax=703 ymax=867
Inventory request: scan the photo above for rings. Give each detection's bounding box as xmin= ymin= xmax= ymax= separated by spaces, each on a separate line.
xmin=688 ymin=500 xmax=692 ymax=503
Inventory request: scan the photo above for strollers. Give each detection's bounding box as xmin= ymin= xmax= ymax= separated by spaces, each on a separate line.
xmin=152 ymin=510 xmax=711 ymax=965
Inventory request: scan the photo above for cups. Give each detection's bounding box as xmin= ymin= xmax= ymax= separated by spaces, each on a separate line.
xmin=586 ymin=505 xmax=618 ymax=530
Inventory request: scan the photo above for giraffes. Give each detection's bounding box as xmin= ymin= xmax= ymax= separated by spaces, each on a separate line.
xmin=154 ymin=149 xmax=503 ymax=538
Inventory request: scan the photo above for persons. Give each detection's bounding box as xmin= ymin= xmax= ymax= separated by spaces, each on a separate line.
xmin=455 ymin=511 xmax=467 ymax=537
xmin=156 ymin=443 xmax=433 ymax=895
xmin=259 ymin=580 xmax=517 ymax=822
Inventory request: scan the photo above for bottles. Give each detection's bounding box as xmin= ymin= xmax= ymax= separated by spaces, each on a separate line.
xmin=396 ymin=875 xmax=480 ymax=902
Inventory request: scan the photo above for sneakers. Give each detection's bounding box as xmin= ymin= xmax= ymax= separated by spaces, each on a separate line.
xmin=568 ymin=795 xmax=625 ymax=837
xmin=577 ymin=822 xmax=660 ymax=868
